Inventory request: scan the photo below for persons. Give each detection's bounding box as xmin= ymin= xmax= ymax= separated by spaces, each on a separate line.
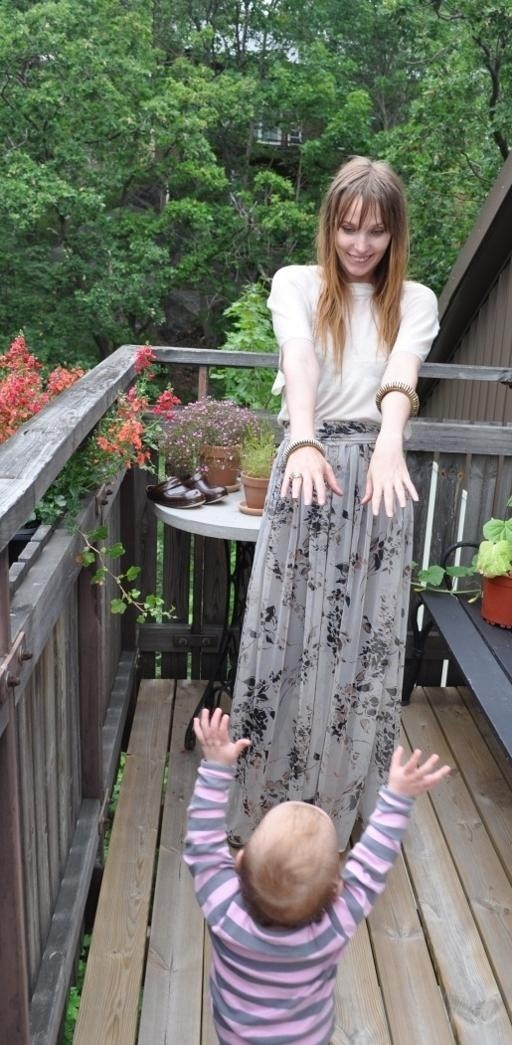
xmin=180 ymin=706 xmax=461 ymax=1045
xmin=223 ymin=154 xmax=444 ymax=860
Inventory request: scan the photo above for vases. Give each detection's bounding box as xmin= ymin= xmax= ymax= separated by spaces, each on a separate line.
xmin=198 ymin=444 xmax=241 ymax=494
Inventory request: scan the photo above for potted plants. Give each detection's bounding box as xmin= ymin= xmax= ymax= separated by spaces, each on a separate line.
xmin=413 ymin=494 xmax=512 ymax=630
xmin=236 ymin=418 xmax=278 ymax=517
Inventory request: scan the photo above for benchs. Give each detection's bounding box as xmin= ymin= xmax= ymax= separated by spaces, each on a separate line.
xmin=401 ymin=539 xmax=512 ymax=763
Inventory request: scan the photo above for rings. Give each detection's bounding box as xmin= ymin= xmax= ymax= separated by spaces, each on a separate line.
xmin=290 ymin=473 xmax=302 ymax=480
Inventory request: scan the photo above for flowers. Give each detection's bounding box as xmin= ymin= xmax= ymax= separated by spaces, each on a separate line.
xmin=159 ymin=394 xmax=258 ymax=472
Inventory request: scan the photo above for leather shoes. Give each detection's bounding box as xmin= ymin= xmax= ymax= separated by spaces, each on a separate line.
xmin=146 ymin=474 xmax=228 ymax=508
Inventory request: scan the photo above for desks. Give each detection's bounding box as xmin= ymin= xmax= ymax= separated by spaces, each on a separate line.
xmin=154 ymin=477 xmax=263 ymax=752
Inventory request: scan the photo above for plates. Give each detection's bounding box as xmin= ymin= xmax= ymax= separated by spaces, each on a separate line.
xmin=223 ymin=479 xmax=242 ymax=493
xmin=240 ymin=501 xmax=263 ymax=514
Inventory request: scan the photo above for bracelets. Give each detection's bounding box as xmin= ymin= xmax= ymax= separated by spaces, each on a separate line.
xmin=374 ymin=382 xmax=421 ymax=419
xmin=279 ymin=438 xmax=329 ymax=473
